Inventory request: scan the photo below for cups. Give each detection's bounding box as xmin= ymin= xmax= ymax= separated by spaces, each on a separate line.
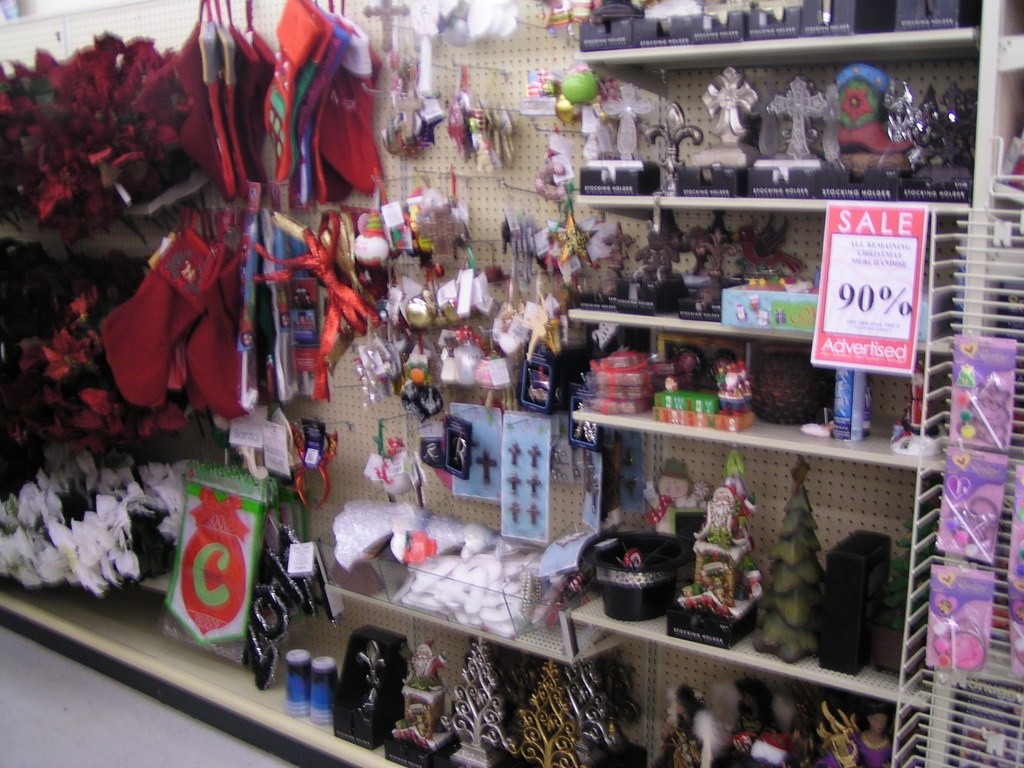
xmin=285 ymin=649 xmax=311 ymax=717
xmin=311 ymin=657 xmax=338 ymax=725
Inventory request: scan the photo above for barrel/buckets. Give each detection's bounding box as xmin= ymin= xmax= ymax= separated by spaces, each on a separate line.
xmin=583 ymin=530 xmax=691 ymax=620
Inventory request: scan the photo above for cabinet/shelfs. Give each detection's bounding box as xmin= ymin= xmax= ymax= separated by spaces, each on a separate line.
xmin=565 ymin=30 xmax=979 ymax=707
xmin=922 ymin=0 xmax=1022 ymax=767
xmin=312 ymin=510 xmax=623 ymax=667
